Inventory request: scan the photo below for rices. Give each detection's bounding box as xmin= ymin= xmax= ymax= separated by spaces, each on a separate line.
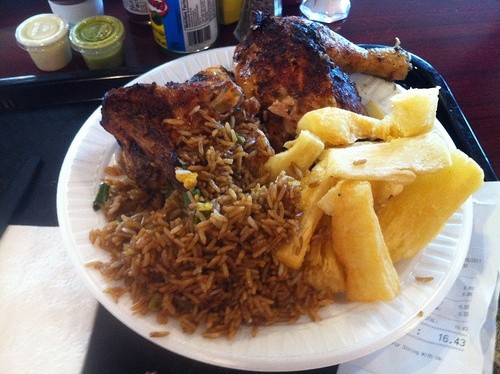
xmin=86 ymin=105 xmax=333 ymax=338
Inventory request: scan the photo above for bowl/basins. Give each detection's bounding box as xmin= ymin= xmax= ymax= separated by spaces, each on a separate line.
xmin=68 ymin=14 xmax=126 ymax=71
xmin=16 ymin=12 xmax=73 ymax=74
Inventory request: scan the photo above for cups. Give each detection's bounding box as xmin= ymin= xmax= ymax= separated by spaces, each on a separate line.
xmin=47 ymin=1 xmax=105 ymax=32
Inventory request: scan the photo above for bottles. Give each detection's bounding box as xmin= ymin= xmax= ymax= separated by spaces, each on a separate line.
xmin=214 ymin=0 xmax=241 ymax=26
xmin=147 ymin=0 xmax=220 ymax=56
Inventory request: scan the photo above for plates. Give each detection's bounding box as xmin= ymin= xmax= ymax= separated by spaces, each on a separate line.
xmin=55 ymin=38 xmax=475 ymax=372
